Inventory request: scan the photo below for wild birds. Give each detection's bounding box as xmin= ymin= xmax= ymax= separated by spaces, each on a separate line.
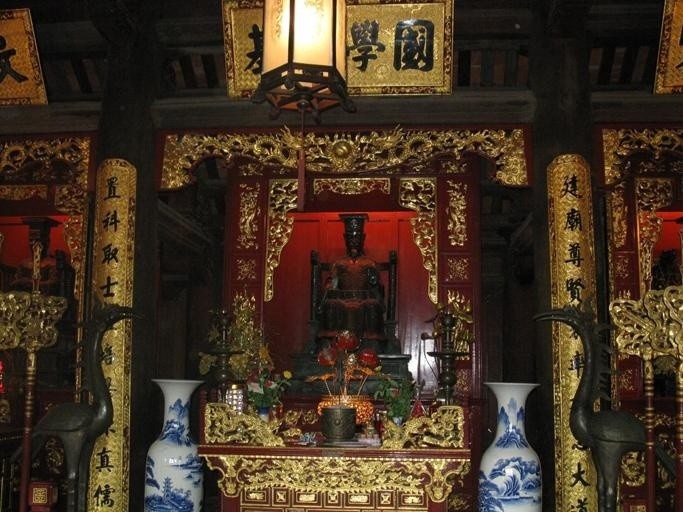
xmin=530 ymin=302 xmax=678 ymax=512
xmin=6 ymin=290 xmax=147 ymax=512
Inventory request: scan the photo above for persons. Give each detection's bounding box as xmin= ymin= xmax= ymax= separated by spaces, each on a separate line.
xmin=313 ymin=212 xmax=385 ymax=370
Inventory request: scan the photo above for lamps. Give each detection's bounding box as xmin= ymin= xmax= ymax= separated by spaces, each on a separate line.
xmin=251 ymin=1 xmax=355 ymax=123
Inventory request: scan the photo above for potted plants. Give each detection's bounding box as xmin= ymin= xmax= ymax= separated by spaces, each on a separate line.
xmin=379 ymin=374 xmax=416 ymax=425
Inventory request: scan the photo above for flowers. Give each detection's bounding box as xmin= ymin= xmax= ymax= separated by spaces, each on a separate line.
xmin=244 ymin=369 xmax=293 ymax=410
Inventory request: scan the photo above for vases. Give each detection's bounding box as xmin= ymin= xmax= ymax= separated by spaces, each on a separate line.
xmin=257 ymin=405 xmax=270 ymax=422
xmin=141 ymin=375 xmax=207 ymax=511
xmin=477 ymin=380 xmax=543 ymax=512
